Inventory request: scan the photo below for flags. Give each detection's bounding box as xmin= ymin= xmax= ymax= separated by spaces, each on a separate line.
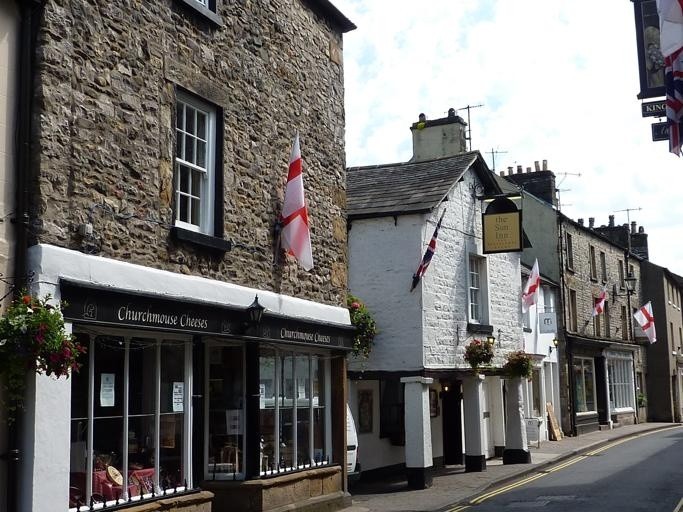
xmin=409 ymin=210 xmax=446 ymax=292
xmin=521 ymin=259 xmax=540 ymax=313
xmin=633 ymin=302 xmax=657 ymax=344
xmin=278 ymin=133 xmax=313 ymax=271
xmin=657 ymin=0 xmax=683 ymax=159
xmin=591 ymin=286 xmax=606 ymax=316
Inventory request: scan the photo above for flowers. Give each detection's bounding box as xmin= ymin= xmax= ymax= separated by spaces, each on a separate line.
xmin=345 ymin=295 xmax=376 ymax=385
xmin=0 ymin=285 xmax=86 ymax=380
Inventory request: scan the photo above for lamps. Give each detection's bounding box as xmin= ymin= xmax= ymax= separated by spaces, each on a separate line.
xmin=612 ymin=271 xmax=636 ymax=303
xmin=463 ymin=339 xmax=533 ymax=382
xmin=549 ymin=337 xmax=559 ymax=353
xmin=486 ymin=334 xmax=495 ymax=345
xmin=246 ymin=294 xmax=265 ymax=328
